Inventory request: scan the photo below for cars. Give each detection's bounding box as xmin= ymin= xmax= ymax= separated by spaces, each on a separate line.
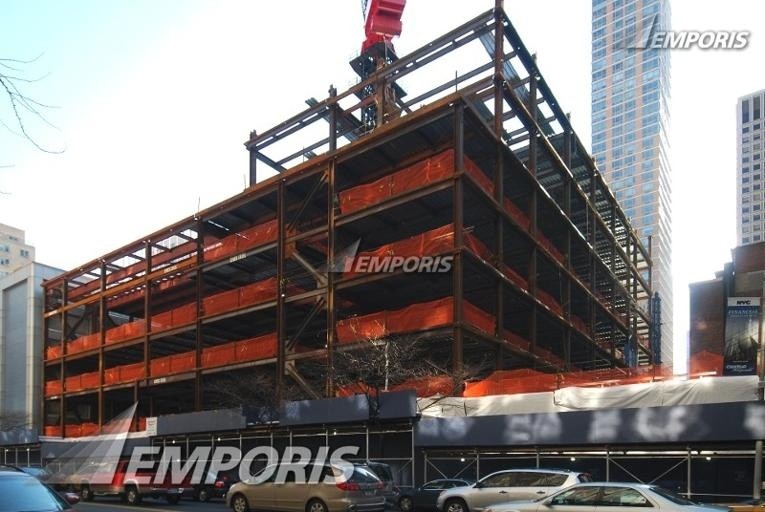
xmin=726 ymin=497 xmax=765 ymax=511
xmin=0 ymin=457 xmax=236 ymax=511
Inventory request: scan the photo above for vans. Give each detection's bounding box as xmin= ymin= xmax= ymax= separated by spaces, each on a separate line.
xmin=222 ymin=456 xmax=387 ymax=512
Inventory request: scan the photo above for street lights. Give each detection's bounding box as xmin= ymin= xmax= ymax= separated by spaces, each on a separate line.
xmin=26 ymin=447 xmax=30 ymax=467
xmin=4 ymin=449 xmax=9 ymax=464
